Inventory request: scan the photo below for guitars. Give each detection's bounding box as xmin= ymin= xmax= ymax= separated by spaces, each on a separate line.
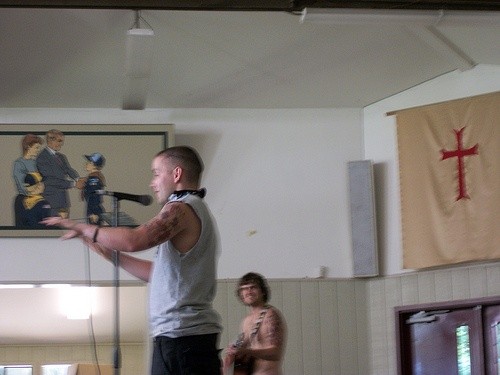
xmin=218 ymin=332 xmax=249 ymax=375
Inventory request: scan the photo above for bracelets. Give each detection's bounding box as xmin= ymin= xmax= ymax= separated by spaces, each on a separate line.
xmin=92 ymin=225 xmax=102 ymax=243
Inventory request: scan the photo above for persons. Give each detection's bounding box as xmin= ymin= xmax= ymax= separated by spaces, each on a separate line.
xmin=39 ymin=146 xmax=224 ymax=375
xmin=222 ymin=272 xmax=288 ymax=375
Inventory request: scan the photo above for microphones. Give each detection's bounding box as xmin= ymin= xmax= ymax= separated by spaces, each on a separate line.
xmin=94 ymin=190 xmax=154 ymax=206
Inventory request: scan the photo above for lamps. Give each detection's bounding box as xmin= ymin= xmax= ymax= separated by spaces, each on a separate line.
xmin=122 ymin=11 xmax=154 ymax=110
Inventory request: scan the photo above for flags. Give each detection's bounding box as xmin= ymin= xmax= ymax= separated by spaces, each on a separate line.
xmin=396 ymin=92 xmax=500 ymax=270
